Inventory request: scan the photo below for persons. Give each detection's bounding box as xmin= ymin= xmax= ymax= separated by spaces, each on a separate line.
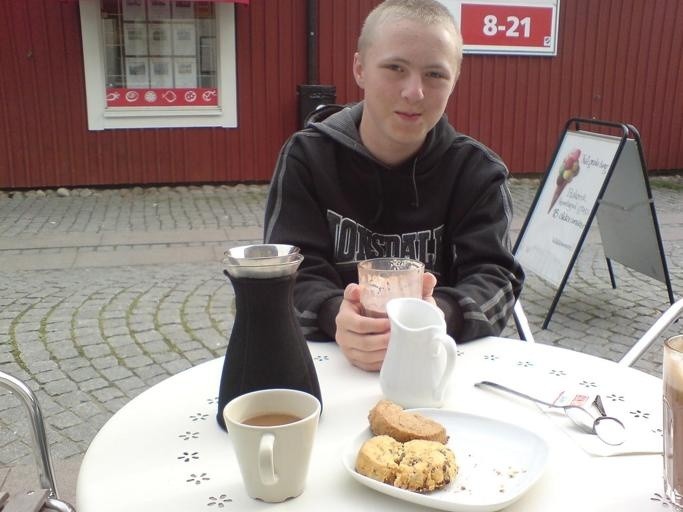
xmin=262 ymin=0 xmax=521 ymax=370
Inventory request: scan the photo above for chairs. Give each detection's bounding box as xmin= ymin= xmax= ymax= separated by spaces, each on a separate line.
xmin=0 ymin=371 xmax=75 ymax=511
xmin=316 ymin=105 xmax=534 ymax=342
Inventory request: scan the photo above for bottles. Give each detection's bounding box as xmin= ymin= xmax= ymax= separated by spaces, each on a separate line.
xmin=214 ymin=241 xmax=324 ymax=428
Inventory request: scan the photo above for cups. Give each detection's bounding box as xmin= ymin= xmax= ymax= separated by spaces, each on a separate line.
xmin=355 ymin=257 xmax=425 ymax=336
xmin=657 ymin=332 xmax=683 ymax=512
xmin=222 ymin=388 xmax=324 ymax=506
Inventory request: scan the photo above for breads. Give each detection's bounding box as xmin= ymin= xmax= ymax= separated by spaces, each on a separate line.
xmin=359 ymin=435 xmax=458 ymax=492
xmin=368 ymin=400 xmax=449 ymax=446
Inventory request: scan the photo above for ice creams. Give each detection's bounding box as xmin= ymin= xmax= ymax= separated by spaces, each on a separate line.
xmin=547 ymin=149 xmax=581 ymax=213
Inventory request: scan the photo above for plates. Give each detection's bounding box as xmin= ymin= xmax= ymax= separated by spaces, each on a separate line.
xmin=344 ymin=406 xmax=546 ymax=512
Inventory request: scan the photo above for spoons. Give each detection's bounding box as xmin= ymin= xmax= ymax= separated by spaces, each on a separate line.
xmin=593 ymin=392 xmax=626 ymax=446
xmin=471 ymin=379 xmax=596 ymax=438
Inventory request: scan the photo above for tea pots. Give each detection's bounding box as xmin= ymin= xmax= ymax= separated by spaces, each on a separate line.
xmin=378 ymin=294 xmax=462 ymax=406
